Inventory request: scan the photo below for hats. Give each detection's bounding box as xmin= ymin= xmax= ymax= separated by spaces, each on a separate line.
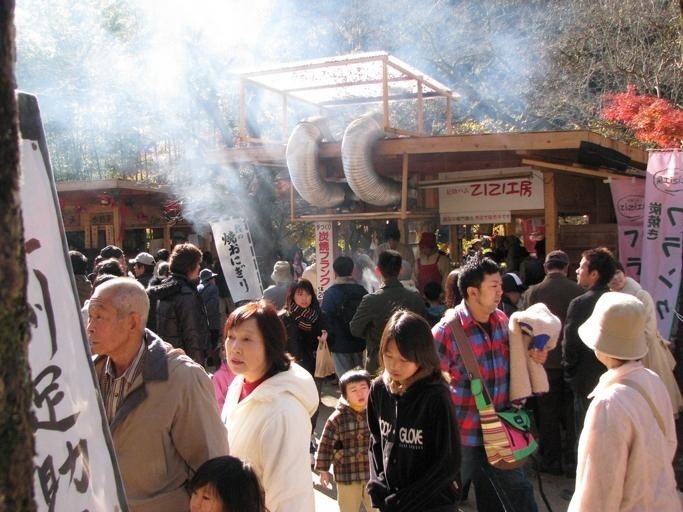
xmin=578 ymin=293 xmax=649 ymax=359
xmin=418 ymin=233 xmax=437 ymax=248
xmin=271 ymin=261 xmax=292 ymax=282
xmin=199 ymin=269 xmax=218 ymax=283
xmin=544 ymin=250 xmax=569 ymax=264
xmin=129 ymin=252 xmax=156 ymax=265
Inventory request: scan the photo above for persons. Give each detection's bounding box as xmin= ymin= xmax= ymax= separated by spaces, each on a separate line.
xmin=262 ymin=261 xmax=293 ymax=310
xmin=518 ymin=249 xmax=587 ymax=476
xmin=399 ymin=260 xmax=418 ymax=291
xmin=85 ymin=275 xmax=228 ymax=512
xmin=292 ymin=254 xmax=306 ymax=281
xmin=219 ymin=301 xmax=320 ymax=512
xmin=302 ymin=261 xmax=319 ymax=295
xmin=194 ymin=267 xmax=222 ymax=338
xmin=349 ymin=249 xmax=427 ymax=379
xmin=607 ymin=260 xmax=683 ymax=420
xmin=187 ymin=456 xmax=266 ymax=512
xmin=315 ymin=368 xmax=373 ymax=512
xmin=147 ymin=243 xmax=208 ymax=367
xmin=410 ymin=233 xmax=450 ymax=297
xmin=373 ymin=227 xmax=414 ymax=269
xmin=68 ymin=245 xmax=168 ymax=308
xmin=210 ymin=339 xmax=241 ymax=411
xmin=275 ymin=278 xmax=328 ymax=467
xmin=561 ymin=246 xmax=614 ymax=504
xmin=422 ymin=282 xmax=445 ymax=316
xmin=445 ymin=234 xmax=546 ymax=318
xmin=566 ymin=291 xmax=679 ymax=512
xmin=430 ymin=257 xmax=547 ymax=512
xmin=366 ymin=311 xmax=463 ymax=512
xmin=321 ymin=256 xmax=369 ymax=378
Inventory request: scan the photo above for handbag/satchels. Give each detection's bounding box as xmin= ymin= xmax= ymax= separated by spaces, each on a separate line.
xmin=446 ymin=310 xmax=539 ymax=468
